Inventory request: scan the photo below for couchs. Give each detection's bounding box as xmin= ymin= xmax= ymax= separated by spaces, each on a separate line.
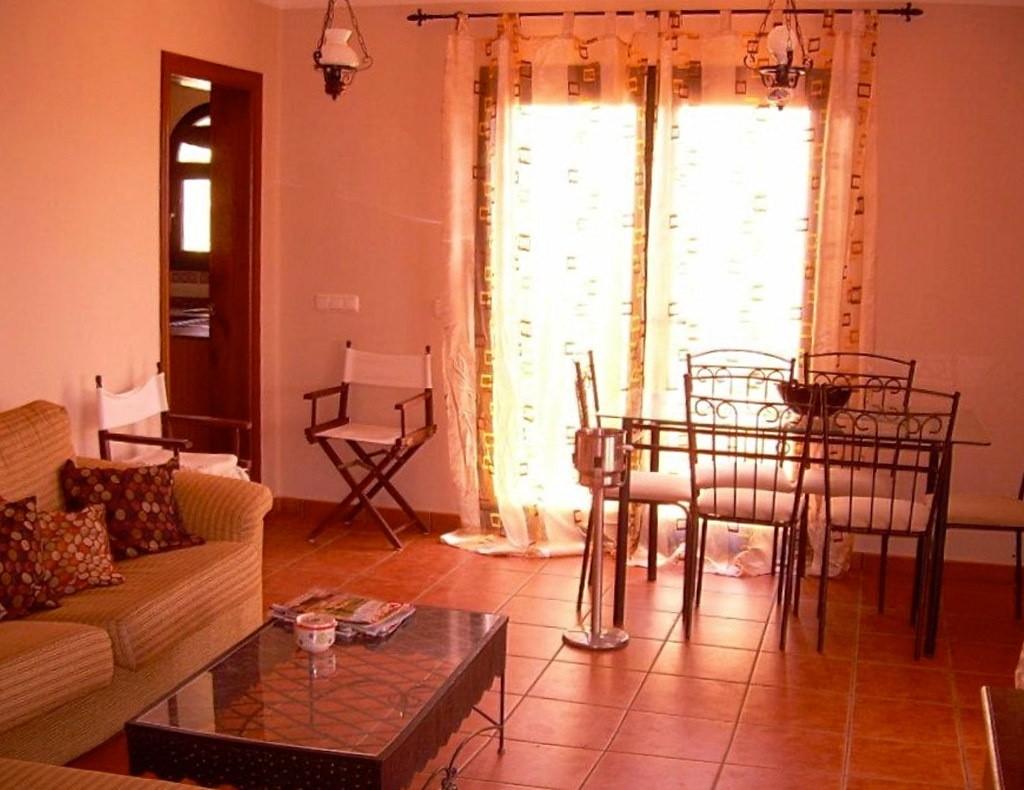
xmin=1 ymin=398 xmax=273 ymax=762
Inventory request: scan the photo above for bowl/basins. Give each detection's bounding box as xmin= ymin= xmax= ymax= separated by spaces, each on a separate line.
xmin=777 ymin=382 xmax=852 ymax=416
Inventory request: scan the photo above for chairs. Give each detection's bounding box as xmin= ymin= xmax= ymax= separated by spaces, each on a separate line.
xmin=94 ymin=363 xmax=253 ymax=482
xmin=304 ymin=339 xmax=438 ymax=549
xmin=573 ymin=347 xmax=961 ymax=662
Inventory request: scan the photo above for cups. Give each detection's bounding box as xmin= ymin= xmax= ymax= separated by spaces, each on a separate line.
xmin=295 ymin=612 xmax=337 ymax=654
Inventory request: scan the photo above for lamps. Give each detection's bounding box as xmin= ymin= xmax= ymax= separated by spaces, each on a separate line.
xmin=742 ymin=1 xmax=815 ymax=112
xmin=313 ymin=1 xmax=373 ymax=101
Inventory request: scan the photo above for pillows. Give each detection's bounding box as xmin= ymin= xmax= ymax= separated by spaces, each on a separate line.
xmin=0 ymin=493 xmax=60 ymax=621
xmin=37 ymin=505 xmax=124 ymax=600
xmin=62 ymin=458 xmax=206 ymax=560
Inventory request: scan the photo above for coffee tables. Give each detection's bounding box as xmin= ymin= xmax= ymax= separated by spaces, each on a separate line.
xmin=127 ymin=592 xmax=509 ymax=790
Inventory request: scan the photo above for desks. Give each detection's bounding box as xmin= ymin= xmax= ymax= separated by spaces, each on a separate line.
xmin=598 ymin=389 xmax=992 ymax=657
